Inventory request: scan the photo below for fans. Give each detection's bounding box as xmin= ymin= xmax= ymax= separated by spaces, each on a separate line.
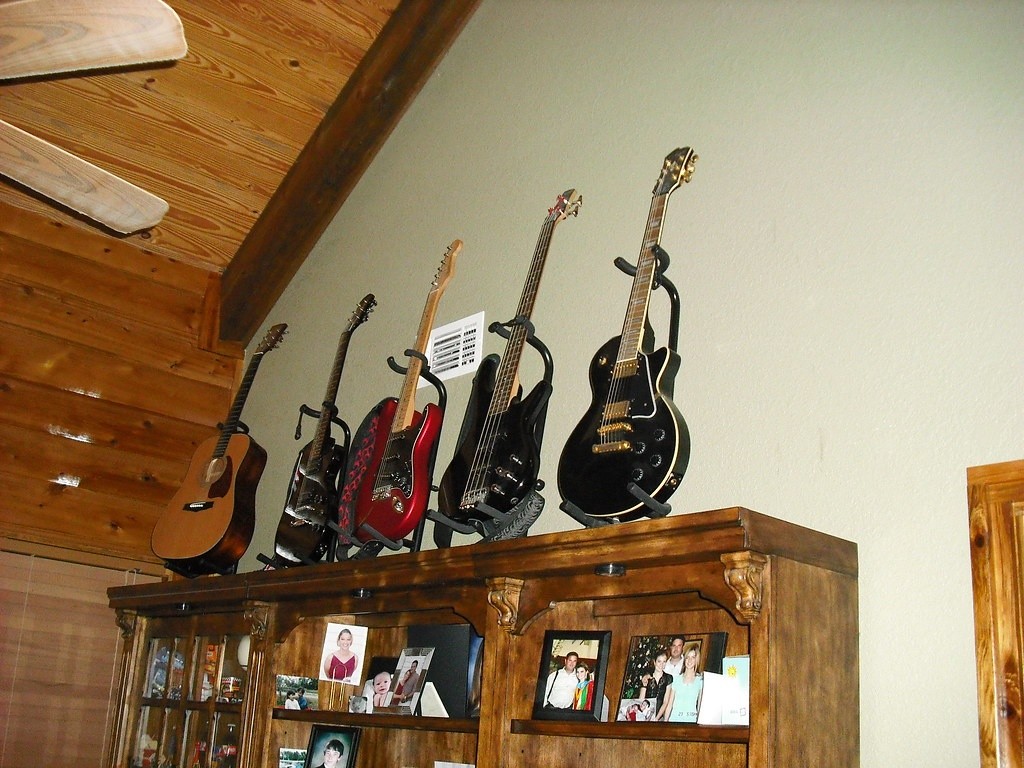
xmin=0 ymin=0 xmax=188 ymax=234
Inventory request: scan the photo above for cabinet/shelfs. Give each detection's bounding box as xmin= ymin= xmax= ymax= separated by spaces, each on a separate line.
xmin=107 ymin=506 xmax=860 ymax=768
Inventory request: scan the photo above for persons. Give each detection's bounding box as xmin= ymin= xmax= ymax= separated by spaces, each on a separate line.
xmin=373 ymin=671 xmax=393 ymax=707
xmin=618 ymin=636 xmax=704 ymax=722
xmin=544 ymin=652 xmax=594 ymax=711
xmin=316 ymin=739 xmax=344 ymax=768
xmin=325 ymin=629 xmax=358 ymax=679
xmin=285 ymin=688 xmax=308 ymax=710
xmin=393 ymin=660 xmax=419 ymax=706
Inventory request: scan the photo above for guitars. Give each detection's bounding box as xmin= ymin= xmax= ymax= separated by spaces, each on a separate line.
xmin=337 ymin=238 xmax=465 ymax=546
xmin=555 ymin=146 xmax=701 ymax=524
xmin=437 ymin=187 xmax=584 ymax=529
xmin=273 ymin=292 xmax=378 ymax=567
xmin=150 ymin=322 xmax=290 ymax=576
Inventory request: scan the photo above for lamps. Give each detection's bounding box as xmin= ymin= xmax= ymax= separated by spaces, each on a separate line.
xmin=351 ymin=588 xmax=372 ymax=599
xmin=175 ymin=603 xmax=193 ymax=611
xmin=595 ymin=563 xmax=626 ymax=577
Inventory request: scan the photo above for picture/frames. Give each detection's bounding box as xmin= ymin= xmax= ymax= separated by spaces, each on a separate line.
xmin=380 ymin=647 xmax=435 ymax=716
xmin=531 ymin=630 xmax=612 ymax=721
xmin=304 ymin=723 xmax=362 ymax=768
xmin=683 ymin=639 xmax=703 ymax=654
